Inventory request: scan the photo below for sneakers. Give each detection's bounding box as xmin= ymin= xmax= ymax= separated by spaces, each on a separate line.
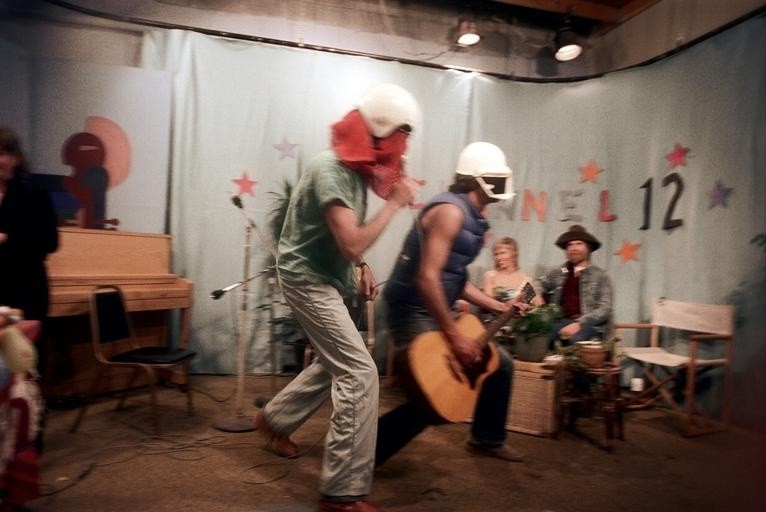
xmin=318 ymin=499 xmax=380 ymax=512
xmin=255 ymin=410 xmax=299 ymax=458
xmin=465 ymin=439 xmax=522 ymax=460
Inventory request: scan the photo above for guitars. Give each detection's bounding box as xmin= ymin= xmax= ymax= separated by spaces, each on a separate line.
xmin=407 ymin=283 xmax=535 ymax=423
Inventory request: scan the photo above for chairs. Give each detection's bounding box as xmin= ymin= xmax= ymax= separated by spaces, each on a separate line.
xmin=610 ymin=293 xmax=737 ymax=437
xmin=69 ymin=283 xmax=198 ymax=442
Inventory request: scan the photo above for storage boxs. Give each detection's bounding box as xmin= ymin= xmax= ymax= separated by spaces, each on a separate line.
xmin=503 ymin=355 xmax=566 ymax=438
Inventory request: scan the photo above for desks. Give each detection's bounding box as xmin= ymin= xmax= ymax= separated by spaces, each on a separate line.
xmin=559 ymin=361 xmax=626 ymax=454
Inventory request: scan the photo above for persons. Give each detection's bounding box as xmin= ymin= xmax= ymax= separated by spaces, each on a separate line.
xmin=531 ymin=223 xmax=615 ymax=416
xmin=252 ymin=82 xmax=421 ymax=511
xmin=0 ymin=125 xmax=57 ymax=457
xmin=372 ymin=141 xmax=526 ymax=478
xmin=482 ymin=235 xmax=535 ymax=323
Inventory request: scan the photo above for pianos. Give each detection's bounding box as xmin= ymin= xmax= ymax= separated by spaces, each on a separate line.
xmin=43 ymin=226 xmax=195 ymax=400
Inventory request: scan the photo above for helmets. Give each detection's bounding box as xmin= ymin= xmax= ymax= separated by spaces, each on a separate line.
xmin=359 ymin=85 xmax=418 ymax=138
xmin=455 ymin=143 xmax=512 ymax=179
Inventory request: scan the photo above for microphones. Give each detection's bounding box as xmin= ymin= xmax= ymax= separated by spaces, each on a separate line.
xmin=232 ymin=196 xmax=255 ymax=224
xmin=211 ymin=282 xmax=243 ymax=299
xmin=397 ymin=155 xmax=414 ymax=209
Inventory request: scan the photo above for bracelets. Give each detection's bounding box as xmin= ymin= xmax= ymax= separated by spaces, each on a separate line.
xmin=359 ymin=262 xmax=366 ymax=267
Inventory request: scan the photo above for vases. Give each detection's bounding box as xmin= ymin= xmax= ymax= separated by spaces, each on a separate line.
xmin=499 ymin=289 xmax=562 ymax=364
xmin=575 ymin=340 xmax=608 ymax=368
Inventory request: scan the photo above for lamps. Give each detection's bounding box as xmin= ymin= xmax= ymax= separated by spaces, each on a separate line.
xmin=550 ymin=0 xmax=585 ymax=65
xmin=452 ymin=0 xmax=482 ymax=48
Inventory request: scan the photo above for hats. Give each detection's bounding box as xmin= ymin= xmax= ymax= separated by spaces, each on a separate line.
xmin=555 ymin=225 xmax=601 ymax=251
